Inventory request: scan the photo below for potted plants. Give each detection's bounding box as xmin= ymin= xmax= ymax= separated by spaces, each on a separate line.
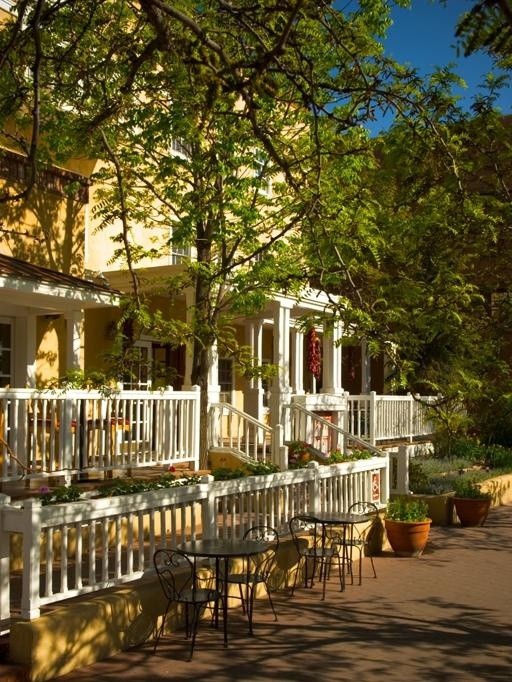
xmin=450 ymin=474 xmax=493 ymax=528
xmin=385 ymin=498 xmax=432 ymax=558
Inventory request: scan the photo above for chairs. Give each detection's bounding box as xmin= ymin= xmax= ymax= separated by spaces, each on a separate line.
xmin=208 ymin=526 xmax=280 ymax=635
xmin=327 ymin=502 xmax=380 ymax=587
xmin=288 ymin=515 xmax=343 ymax=600
xmin=150 ymin=548 xmax=227 ymax=661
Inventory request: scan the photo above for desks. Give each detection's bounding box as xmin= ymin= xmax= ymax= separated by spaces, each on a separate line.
xmin=174 ymin=539 xmax=270 ymax=649
xmin=300 ymin=511 xmax=371 ymax=592
